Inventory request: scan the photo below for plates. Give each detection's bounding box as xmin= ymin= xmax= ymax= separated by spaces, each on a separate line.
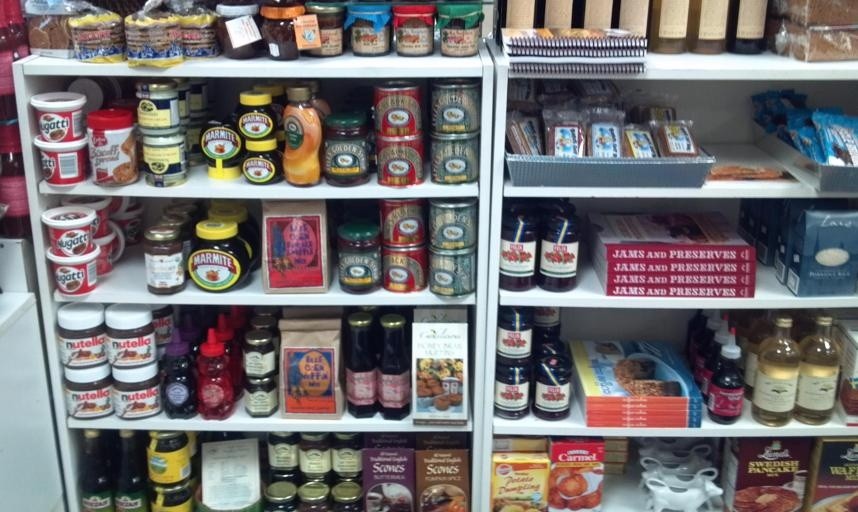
xmin=366 ymin=504 xmax=390 ymax=512
xmin=733 ymin=484 xmax=802 ymax=511
xmin=784 ymin=482 xmax=807 ymax=498
xmin=810 ymin=492 xmax=853 ymax=512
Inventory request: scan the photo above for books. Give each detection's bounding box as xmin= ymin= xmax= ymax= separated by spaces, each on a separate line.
xmin=570 ymin=335 xmax=704 ymax=433
xmin=582 ymin=207 xmax=757 ymax=298
xmin=497 ymin=22 xmax=649 ymax=79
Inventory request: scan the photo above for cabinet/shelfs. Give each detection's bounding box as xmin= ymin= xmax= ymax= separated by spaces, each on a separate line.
xmin=480 ymin=34 xmax=857 ymax=511
xmin=10 ymin=38 xmax=494 ymax=512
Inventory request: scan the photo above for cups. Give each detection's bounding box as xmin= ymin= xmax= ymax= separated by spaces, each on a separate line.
xmin=793 ymin=469 xmax=808 ymax=497
xmin=367 ymin=492 xmax=384 ymax=508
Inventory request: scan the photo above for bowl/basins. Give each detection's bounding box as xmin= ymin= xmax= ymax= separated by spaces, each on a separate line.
xmin=626 ymin=353 xmax=690 ymax=403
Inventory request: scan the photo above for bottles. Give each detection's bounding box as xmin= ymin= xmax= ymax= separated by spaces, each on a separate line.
xmin=281 ymin=81 xmax=333 ymax=187
xmin=686 ymin=309 xmax=840 ymax=426
xmin=159 ymin=304 xmax=243 ymax=421
xmin=494 ymin=308 xmax=571 ymax=420
xmin=505 ymin=0 xmax=773 ymax=57
xmin=342 ymin=307 xmax=410 ymax=419
xmin=501 ymin=198 xmax=581 ymax=291
xmin=79 ymin=427 xmax=148 ymax=512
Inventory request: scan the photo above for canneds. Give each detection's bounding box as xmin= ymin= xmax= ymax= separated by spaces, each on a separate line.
xmin=133 ymin=77 xmax=209 ymax=188
xmin=145 ymin=201 xmax=199 ymax=296
xmin=263 ymin=430 xmax=365 ymax=511
xmin=335 ymin=197 xmax=478 ymax=296
xmin=500 ymin=204 xmax=578 ymax=291
xmin=141 ymin=430 xmax=199 ymax=512
xmin=215 ymin=2 xmax=483 ymax=61
xmin=55 ymin=304 xmax=177 ymax=419
xmin=321 ymin=82 xmax=479 ymax=185
xmin=243 ymin=308 xmax=278 ymax=419
xmin=495 ymin=305 xmax=572 ymax=419
